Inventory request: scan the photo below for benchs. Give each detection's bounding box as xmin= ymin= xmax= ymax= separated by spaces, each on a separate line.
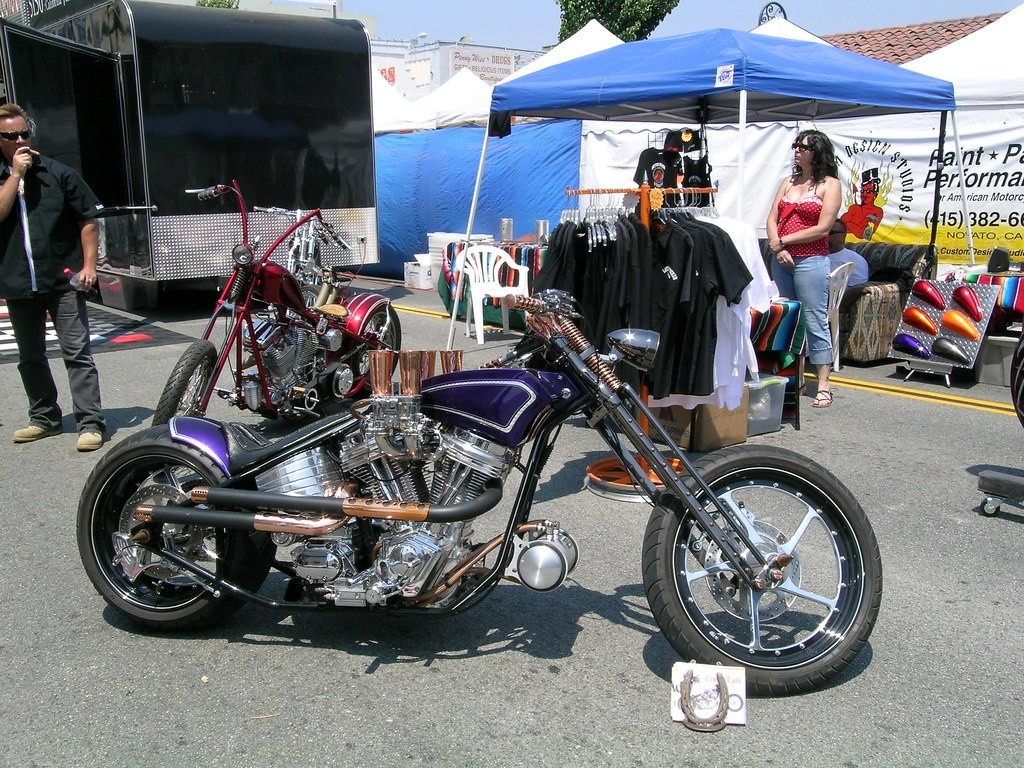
xmin=755 ymin=237 xmax=941 ymax=366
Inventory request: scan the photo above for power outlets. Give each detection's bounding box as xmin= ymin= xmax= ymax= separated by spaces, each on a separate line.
xmin=357 ymin=236 xmax=368 ymax=245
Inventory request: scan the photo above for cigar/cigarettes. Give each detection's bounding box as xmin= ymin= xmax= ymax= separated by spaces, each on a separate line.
xmin=29 ymin=148 xmax=40 ymax=154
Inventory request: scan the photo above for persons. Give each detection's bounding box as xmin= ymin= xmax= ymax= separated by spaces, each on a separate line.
xmin=0 ymin=102 xmax=107 ymax=450
xmin=828 ymin=218 xmax=869 ymax=287
xmin=766 ymin=129 xmax=842 ymax=408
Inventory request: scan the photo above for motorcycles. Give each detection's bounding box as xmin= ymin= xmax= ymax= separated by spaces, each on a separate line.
xmin=151 ymin=179 xmax=402 ymax=429
xmin=76 ymin=287 xmax=883 ymax=699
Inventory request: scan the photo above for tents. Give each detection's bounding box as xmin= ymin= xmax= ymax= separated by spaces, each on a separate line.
xmin=370 ymin=62 xmax=436 ymax=134
xmin=413 ymin=63 xmax=497 ymax=130
xmin=492 ymin=17 xmax=628 ymax=84
xmin=446 ymin=26 xmax=978 ymax=350
xmin=897 ymin=0 xmax=1024 ymax=99
xmin=749 ymin=15 xmax=835 ymax=46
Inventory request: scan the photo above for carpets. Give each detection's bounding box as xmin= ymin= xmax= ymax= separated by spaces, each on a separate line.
xmin=0 ymin=297 xmax=203 ymax=365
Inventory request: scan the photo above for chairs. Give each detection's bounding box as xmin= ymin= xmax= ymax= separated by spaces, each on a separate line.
xmin=798 ymin=262 xmax=857 ymax=394
xmin=455 ymin=245 xmax=531 ymax=344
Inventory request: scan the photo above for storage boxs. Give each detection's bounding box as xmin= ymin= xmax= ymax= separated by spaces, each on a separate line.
xmin=404 ymin=229 xmax=496 ymax=292
xmin=632 ymin=367 xmax=791 ymax=456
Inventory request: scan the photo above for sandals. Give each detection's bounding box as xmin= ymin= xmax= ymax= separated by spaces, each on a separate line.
xmin=811 ymin=389 xmax=834 ymax=408
xmin=799 ymin=383 xmax=809 ymax=396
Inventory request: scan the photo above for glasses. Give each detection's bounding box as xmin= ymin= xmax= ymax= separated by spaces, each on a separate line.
xmin=0 ymin=130 xmax=32 ymax=141
xmin=792 ymin=142 xmax=812 ymax=152
xmin=829 ymin=230 xmax=843 ymax=236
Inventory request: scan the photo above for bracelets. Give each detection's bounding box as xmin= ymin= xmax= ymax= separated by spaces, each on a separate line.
xmin=779 ymin=236 xmax=785 ymax=247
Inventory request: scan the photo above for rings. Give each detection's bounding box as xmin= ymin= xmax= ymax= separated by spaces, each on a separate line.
xmin=779 ymin=261 xmax=783 ymax=264
xmin=783 ymin=258 xmax=788 ymax=264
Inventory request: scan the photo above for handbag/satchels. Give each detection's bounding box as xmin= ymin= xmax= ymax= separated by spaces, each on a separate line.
xmin=671 ymin=660 xmax=746 ymax=733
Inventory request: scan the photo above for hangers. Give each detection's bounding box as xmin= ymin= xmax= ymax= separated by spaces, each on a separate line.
xmin=550 ymin=186 xmax=721 ymax=254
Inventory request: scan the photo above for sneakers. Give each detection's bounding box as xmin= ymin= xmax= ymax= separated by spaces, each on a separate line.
xmin=75 ymin=431 xmax=103 ymax=450
xmin=13 ymin=425 xmax=63 ymax=442
